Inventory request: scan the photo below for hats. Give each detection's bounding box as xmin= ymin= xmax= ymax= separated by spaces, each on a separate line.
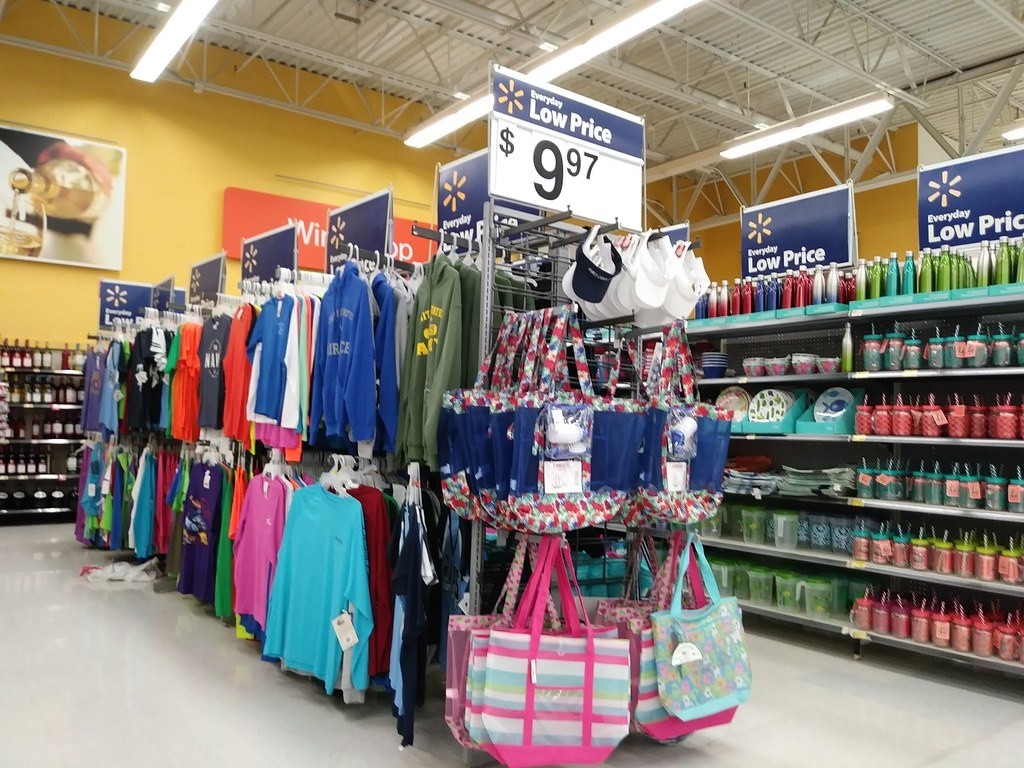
xmin=562 ymin=224 xmax=711 ymax=327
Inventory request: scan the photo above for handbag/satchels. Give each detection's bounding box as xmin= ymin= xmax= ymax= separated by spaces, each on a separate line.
xmin=445 ymin=534 xmax=753 ymax=768
xmin=437 ymin=304 xmax=735 ymax=536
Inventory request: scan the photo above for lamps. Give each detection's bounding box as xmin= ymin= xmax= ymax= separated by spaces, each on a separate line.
xmin=998 ymin=120 xmax=1024 ymax=141
xmin=129 ymin=0 xmax=219 ymax=84
xmin=644 ymin=91 xmax=895 ymax=187
xmin=402 ymin=0 xmax=703 ymax=149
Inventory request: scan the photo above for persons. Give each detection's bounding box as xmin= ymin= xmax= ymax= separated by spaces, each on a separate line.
xmin=0 ymin=128 xmax=112 ymax=203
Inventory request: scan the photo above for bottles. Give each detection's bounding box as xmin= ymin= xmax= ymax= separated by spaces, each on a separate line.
xmin=840 ymin=320 xmax=854 ymax=371
xmin=0 ymin=483 xmax=78 ymax=509
xmin=0 ymin=338 xmax=94 ymax=370
xmin=0 ymin=444 xmax=83 ymax=475
xmin=6 ymin=407 xmax=85 ymax=439
xmin=0 ymin=373 xmax=85 ymax=404
xmin=695 ymin=262 xmax=857 ymax=320
xmin=856 ymin=233 xmax=1024 ymax=300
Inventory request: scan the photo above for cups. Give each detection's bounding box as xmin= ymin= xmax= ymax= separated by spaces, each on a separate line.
xmin=850 ymin=597 xmax=1024 ymax=663
xmin=855 ymin=405 xmax=1024 ymax=440
xmin=856 ymin=468 xmax=1024 ymax=514
xmin=852 ymin=530 xmax=1024 ymax=586
xmin=863 ymin=332 xmax=1024 ymax=371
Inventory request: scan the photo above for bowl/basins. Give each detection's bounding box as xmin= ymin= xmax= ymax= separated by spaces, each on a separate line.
xmin=701 ymin=352 xmax=729 ymax=378
xmin=742 ymin=353 xmax=841 ymax=376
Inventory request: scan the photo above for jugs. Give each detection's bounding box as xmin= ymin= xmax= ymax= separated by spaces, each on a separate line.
xmin=656 ymin=504 xmax=854 ymax=556
xmin=695 ymin=556 xmax=867 ymax=620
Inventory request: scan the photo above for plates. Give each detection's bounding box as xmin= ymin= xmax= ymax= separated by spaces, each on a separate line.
xmin=814 ymin=387 xmax=853 ymax=422
xmin=749 ymin=389 xmax=797 ymax=422
xmin=715 ymin=386 xmax=753 ymax=422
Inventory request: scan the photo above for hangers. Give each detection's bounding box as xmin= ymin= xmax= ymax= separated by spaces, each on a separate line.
xmin=435 ymin=225 xmax=540 ymax=291
xmin=92 ymin=243 xmax=426 ymax=354
xmin=83 ymin=427 xmax=425 ymax=507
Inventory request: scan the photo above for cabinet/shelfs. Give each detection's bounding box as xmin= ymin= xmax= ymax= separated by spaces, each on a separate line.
xmin=848 ymin=280 xmax=1024 ymax=677
xmin=0 ymin=368 xmax=84 ymax=514
xmin=689 ymin=302 xmax=849 ymax=636
